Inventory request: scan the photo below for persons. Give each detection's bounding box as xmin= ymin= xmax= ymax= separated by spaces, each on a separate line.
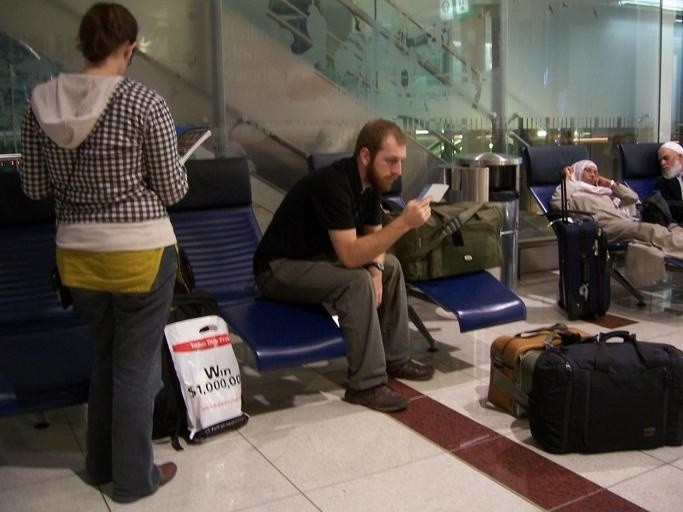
xmin=251 ymin=118 xmax=435 ymax=414
xmin=268 ymin=0 xmax=312 ymax=55
xmin=17 ymin=0 xmax=190 ymax=503
xmin=642 ymin=140 xmax=683 ymax=229
xmin=549 ymin=160 xmax=683 ymax=262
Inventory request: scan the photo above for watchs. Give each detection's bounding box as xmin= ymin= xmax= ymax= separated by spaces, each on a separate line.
xmin=365 ymin=261 xmax=385 ymax=273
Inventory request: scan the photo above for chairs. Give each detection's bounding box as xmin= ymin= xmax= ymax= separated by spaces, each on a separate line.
xmin=0 ymin=164 xmax=91 ymax=433
xmin=522 ymin=143 xmax=683 ymax=310
xmin=165 ymin=154 xmax=347 ymax=377
xmin=618 ymin=144 xmax=666 ymax=201
xmin=379 ymin=175 xmax=529 ymax=353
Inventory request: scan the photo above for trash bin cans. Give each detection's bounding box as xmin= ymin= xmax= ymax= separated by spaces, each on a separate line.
xmin=450 ymin=151 xmax=522 ymax=295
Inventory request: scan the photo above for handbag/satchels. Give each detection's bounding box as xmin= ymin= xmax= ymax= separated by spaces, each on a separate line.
xmin=382 ymin=200 xmax=504 ymax=284
xmin=151 ymin=291 xmax=250 ymax=453
xmin=486 ymin=323 xmax=683 ymax=458
xmin=48 ymin=243 xmax=74 ymax=312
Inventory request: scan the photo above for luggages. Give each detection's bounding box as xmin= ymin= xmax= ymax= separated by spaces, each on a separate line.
xmin=556 ymin=213 xmax=612 ymax=321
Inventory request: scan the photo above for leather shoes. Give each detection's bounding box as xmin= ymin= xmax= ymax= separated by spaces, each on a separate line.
xmin=343 ymin=383 xmax=409 ymax=413
xmin=385 ymin=360 xmax=435 ymax=382
xmin=156 ymin=461 xmax=177 ymax=489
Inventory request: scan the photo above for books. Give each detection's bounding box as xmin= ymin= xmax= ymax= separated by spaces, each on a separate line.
xmin=177 ymin=127 xmax=212 ymax=165
